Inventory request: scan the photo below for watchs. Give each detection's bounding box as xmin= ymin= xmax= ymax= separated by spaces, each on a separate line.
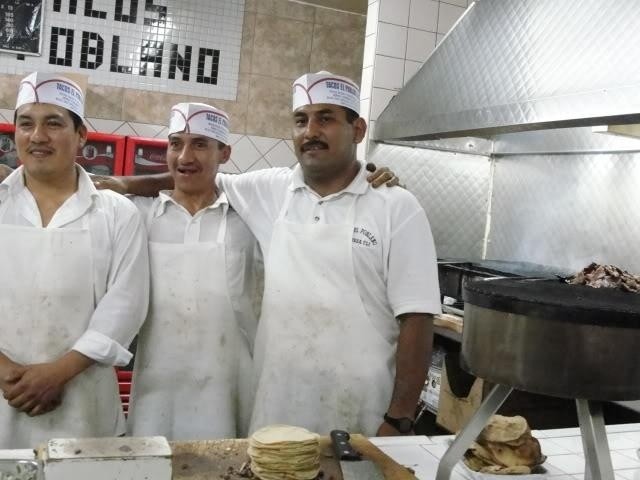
xmin=383 ymin=412 xmax=414 ymax=433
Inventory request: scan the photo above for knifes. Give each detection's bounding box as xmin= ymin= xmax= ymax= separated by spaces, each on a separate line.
xmin=331 ymin=430 xmax=387 ymax=480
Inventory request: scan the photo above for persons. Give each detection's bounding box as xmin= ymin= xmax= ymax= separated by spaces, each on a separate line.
xmin=88 ymin=70 xmax=443 ymax=441
xmin=1 ymin=102 xmax=408 ymax=439
xmin=0 ymin=72 xmax=151 ymax=450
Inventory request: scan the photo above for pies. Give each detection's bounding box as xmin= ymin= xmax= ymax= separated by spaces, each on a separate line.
xmin=246 ymin=424 xmax=322 ymax=480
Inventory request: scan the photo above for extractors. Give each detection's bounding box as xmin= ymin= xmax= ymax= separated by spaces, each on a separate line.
xmin=374 ymin=0 xmax=640 ymax=156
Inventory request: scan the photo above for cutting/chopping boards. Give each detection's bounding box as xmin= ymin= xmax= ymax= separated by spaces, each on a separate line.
xmin=33 ymin=434 xmax=418 ymax=479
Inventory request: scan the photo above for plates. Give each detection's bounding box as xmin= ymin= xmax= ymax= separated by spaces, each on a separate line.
xmin=446 ymin=439 xmax=548 ymax=480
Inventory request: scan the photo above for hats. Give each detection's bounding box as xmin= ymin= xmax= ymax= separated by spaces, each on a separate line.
xmin=167 ymin=102 xmax=230 ymax=146
xmin=15 ymin=72 xmax=86 ymax=124
xmin=291 ymin=71 xmax=361 ymax=116
